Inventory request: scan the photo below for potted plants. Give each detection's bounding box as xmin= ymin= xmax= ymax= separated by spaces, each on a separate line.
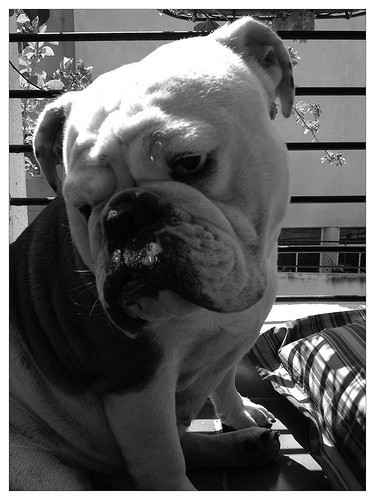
xmin=326 ymin=256 xmax=344 ymax=273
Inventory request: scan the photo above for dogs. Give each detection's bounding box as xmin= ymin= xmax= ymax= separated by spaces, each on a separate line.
xmin=9 ymin=15 xmax=295 ymax=492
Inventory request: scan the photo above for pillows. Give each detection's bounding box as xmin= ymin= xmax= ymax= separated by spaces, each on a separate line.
xmin=246 ymin=308 xmax=366 ymax=491
